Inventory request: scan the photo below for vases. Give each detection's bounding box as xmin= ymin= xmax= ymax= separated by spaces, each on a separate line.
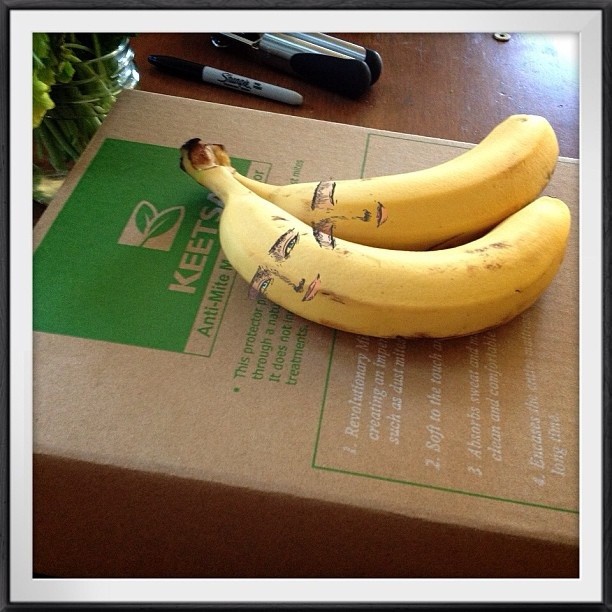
xmin=33 ymin=35 xmax=143 ymax=207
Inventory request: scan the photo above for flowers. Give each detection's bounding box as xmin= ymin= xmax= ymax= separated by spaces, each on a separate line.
xmin=33 ymin=33 xmax=130 ymax=175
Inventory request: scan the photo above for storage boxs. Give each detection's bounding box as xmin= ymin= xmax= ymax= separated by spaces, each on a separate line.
xmin=31 ymin=90 xmax=580 ymax=578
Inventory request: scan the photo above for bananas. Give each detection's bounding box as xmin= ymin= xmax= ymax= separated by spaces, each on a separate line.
xmin=178 ymin=111 xmax=572 ymax=343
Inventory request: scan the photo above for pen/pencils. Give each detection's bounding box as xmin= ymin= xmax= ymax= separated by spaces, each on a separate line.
xmin=147 ymin=55 xmax=303 ymax=105
